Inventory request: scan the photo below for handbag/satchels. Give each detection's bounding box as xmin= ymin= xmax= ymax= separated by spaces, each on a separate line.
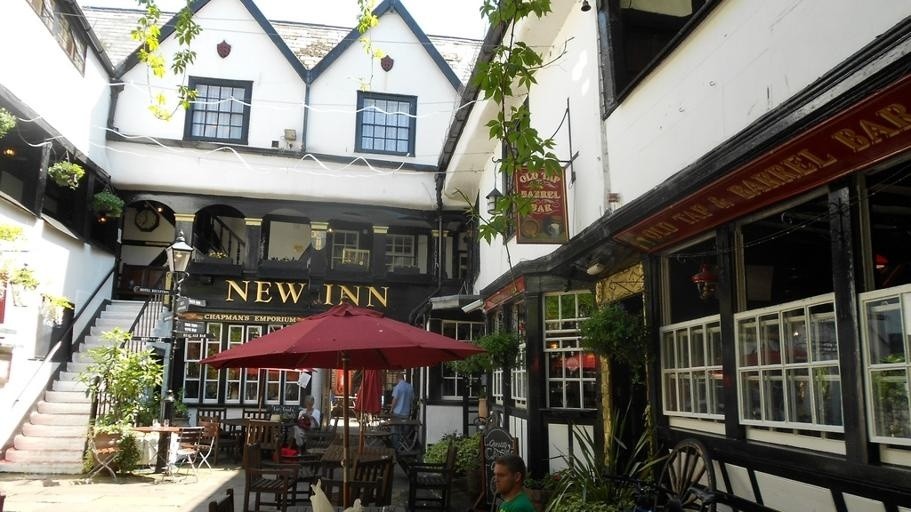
xmin=296 ymin=413 xmax=318 ymax=430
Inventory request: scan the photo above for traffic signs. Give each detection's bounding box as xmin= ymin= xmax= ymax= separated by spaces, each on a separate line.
xmin=124 ymin=285 xmax=217 ymax=347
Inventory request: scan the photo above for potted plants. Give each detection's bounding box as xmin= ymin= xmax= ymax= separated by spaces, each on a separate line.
xmin=48 ymin=151 xmax=85 ymax=191
xmin=89 ymin=175 xmax=124 ymax=224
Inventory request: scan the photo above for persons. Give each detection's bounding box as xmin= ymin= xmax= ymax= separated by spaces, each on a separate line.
xmin=389 ymin=374 xmax=414 ymax=447
xmin=286 ymin=395 xmax=320 ymax=456
xmin=493 ymin=455 xmax=537 ymax=512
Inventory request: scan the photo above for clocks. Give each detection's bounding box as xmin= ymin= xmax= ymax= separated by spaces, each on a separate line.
xmin=135 ymin=207 xmax=159 ymax=232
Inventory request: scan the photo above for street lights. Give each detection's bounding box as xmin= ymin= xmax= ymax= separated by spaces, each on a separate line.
xmin=162 ymin=229 xmax=194 ymax=426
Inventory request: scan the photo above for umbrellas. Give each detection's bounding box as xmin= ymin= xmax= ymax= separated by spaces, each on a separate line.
xmin=355 ymin=370 xmax=384 ymax=453
xmin=198 ymin=303 xmax=488 ymax=512
